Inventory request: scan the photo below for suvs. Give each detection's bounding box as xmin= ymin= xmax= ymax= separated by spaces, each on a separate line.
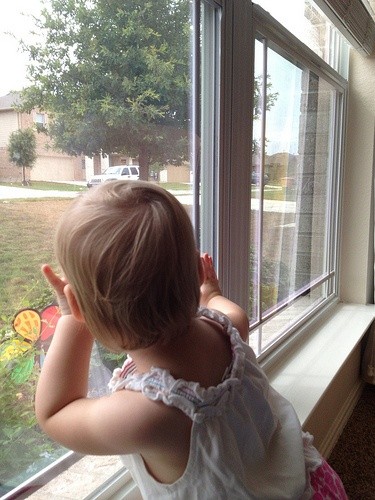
xmin=87 ymin=165 xmax=141 ymax=189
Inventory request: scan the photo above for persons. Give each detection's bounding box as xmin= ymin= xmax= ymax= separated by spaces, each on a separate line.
xmin=33 ymin=177 xmax=355 ymax=500
